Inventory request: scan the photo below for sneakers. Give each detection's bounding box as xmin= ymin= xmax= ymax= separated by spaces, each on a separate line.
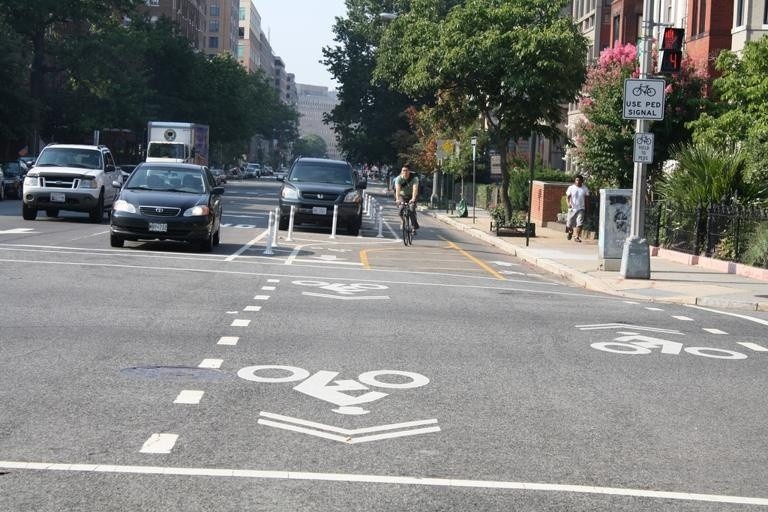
xmin=567 ymin=231 xmax=572 ymax=240
xmin=575 ymin=237 xmax=581 ymax=242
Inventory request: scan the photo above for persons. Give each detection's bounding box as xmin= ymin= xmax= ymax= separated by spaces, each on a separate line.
xmin=394 ymin=165 xmax=420 ymax=236
xmin=565 ymin=175 xmax=591 ymax=242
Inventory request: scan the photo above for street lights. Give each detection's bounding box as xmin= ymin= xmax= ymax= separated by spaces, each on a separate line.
xmin=470 ymin=131 xmax=479 ymax=223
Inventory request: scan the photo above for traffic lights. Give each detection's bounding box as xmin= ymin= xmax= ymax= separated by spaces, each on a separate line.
xmin=658 ymin=27 xmax=685 ymax=73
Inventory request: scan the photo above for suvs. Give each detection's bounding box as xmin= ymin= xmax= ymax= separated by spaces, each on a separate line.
xmin=276 ymin=154 xmax=367 ymax=235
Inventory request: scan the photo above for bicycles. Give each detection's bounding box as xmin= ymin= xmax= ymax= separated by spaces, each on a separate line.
xmin=393 ymin=200 xmax=415 ymax=246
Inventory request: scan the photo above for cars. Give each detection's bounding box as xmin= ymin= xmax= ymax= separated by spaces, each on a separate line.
xmin=0 ymin=121 xmax=288 ymax=220
xmin=105 ymin=161 xmax=225 ymax=249
